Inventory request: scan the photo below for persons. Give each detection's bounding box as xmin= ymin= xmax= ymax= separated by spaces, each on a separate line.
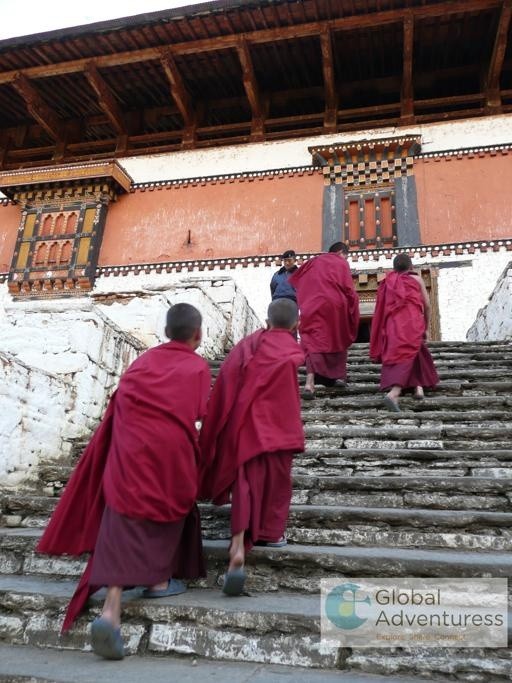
xmin=198 ymin=297 xmax=307 ymax=596
xmin=288 ymin=242 xmax=360 ymax=400
xmin=34 ymin=302 xmax=211 ymax=660
xmin=368 ymin=254 xmax=440 ymax=412
xmin=270 ymin=250 xmax=298 ymax=342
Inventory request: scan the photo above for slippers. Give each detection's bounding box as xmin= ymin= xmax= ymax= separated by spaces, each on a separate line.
xmin=222 ymin=573 xmax=244 ymax=596
xmin=91 ymin=620 xmax=125 ymax=660
xmin=268 ymin=534 xmax=287 ymax=547
xmin=143 ymin=578 xmax=186 ymax=598
xmin=336 ymin=380 xmax=349 ymax=387
xmin=383 ymin=396 xmax=400 ymax=412
xmin=300 ymin=392 xmax=317 ymax=399
